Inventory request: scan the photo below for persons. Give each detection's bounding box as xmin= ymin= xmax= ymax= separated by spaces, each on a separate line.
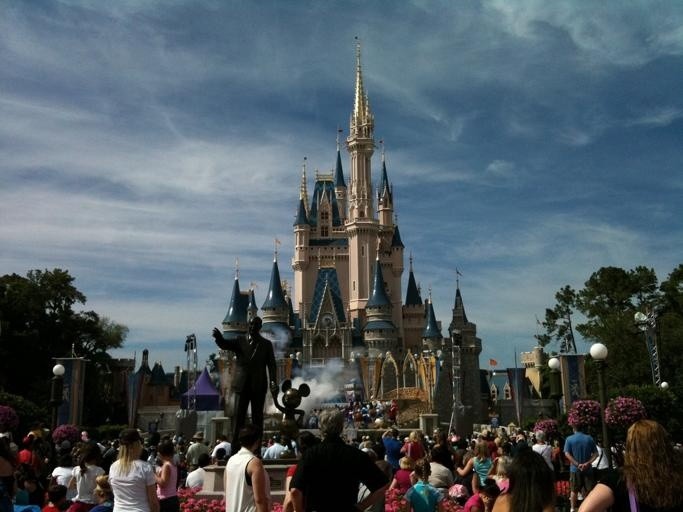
xmin=575 ymin=420 xmax=682 ymax=512
xmin=1 ymin=394 xmax=612 ymax=512
xmin=210 ymin=314 xmax=278 ymax=457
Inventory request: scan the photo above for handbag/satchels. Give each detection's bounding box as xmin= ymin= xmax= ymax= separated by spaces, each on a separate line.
xmin=591 ymin=467 xmax=598 ymax=476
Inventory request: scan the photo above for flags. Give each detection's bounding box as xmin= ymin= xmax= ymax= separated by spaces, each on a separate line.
xmin=489 ymin=359 xmax=497 ymax=367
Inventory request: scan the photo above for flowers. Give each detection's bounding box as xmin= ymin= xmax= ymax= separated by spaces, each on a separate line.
xmin=532 ymin=418 xmax=559 ymax=440
xmin=604 ymin=396 xmax=647 ymax=428
xmin=0 ymin=405 xmax=19 ymax=432
xmin=52 ymin=423 xmax=77 ymax=443
xmin=568 ymin=401 xmax=601 ymax=428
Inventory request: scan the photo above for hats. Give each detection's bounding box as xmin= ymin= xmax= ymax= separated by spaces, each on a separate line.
xmin=192 ymin=434 xmax=202 ymax=439
xmin=119 ymin=428 xmax=142 ymax=445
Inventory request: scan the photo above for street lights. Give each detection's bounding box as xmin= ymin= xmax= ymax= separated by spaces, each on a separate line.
xmin=589 ymin=341 xmax=612 ymax=475
xmin=49 ymin=363 xmax=65 ymax=431
xmin=406 ymin=341 xmax=444 ymax=414
xmin=548 ymin=358 xmax=563 ymax=427
